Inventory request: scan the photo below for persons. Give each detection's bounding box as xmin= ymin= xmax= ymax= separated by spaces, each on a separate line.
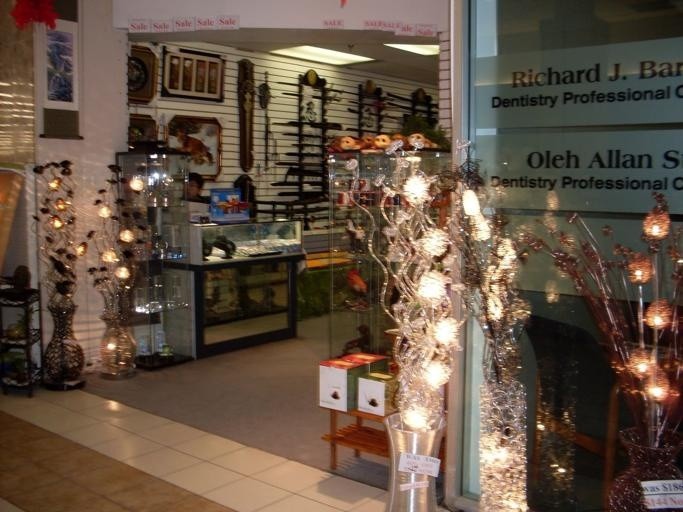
xmin=176 ymin=171 xmax=213 ymax=206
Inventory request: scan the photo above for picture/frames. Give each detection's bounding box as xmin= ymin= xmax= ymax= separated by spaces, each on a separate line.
xmin=127 ymin=39 xmax=227 ymax=181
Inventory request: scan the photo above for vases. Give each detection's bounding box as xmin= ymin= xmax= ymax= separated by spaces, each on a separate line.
xmin=376 ymin=405 xmax=449 ymax=512
xmin=474 ymin=376 xmax=528 ymax=512
xmin=601 ymin=425 xmax=682 ymax=511
xmin=38 ymin=301 xmax=140 ymax=388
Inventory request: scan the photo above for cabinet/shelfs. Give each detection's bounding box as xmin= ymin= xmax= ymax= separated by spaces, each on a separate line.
xmin=0 ymin=287 xmax=40 ymax=399
xmin=314 ymin=403 xmax=390 ymax=476
xmin=158 ymin=253 xmax=307 ymax=361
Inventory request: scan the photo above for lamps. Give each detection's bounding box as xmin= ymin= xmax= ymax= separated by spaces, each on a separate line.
xmin=27 ymin=151 xmax=156 ymax=360
